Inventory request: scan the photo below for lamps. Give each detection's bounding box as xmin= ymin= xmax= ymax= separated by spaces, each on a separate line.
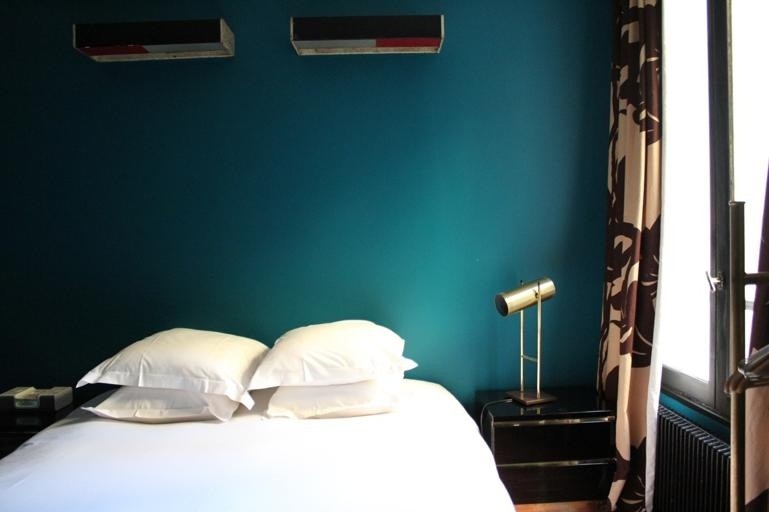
xmin=72 ymin=17 xmax=235 ymax=62
xmin=492 ymin=276 xmax=559 ymax=407
xmin=291 ymin=15 xmax=445 ymax=56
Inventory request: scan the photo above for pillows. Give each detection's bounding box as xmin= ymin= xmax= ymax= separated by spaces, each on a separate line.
xmin=76 ymin=321 xmax=271 ymax=410
xmin=77 ymin=384 xmax=241 ymax=423
xmin=245 ymin=320 xmax=417 ymax=393
xmin=262 ymin=384 xmax=395 ymax=423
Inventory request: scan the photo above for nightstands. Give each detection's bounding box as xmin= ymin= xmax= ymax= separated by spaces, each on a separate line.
xmin=472 ymin=389 xmax=617 ymax=506
xmin=0 ymin=385 xmax=75 ymax=458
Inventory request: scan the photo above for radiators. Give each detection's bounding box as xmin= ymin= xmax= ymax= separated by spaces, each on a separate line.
xmin=653 ymin=403 xmax=730 ymax=512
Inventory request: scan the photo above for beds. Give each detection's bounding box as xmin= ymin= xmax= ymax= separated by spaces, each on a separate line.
xmin=0 ymin=379 xmax=520 ymax=512
